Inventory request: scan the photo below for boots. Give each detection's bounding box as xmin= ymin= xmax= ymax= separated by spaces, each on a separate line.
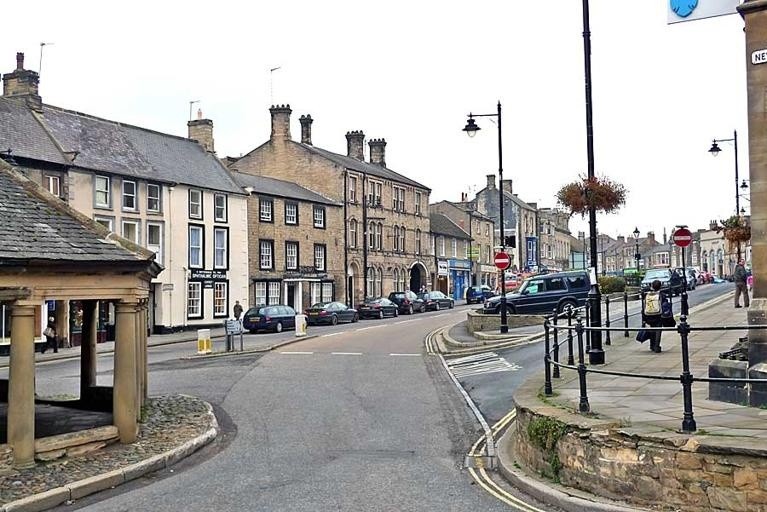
xmin=638 ymin=268 xmax=685 ymax=298
xmin=481 ymin=270 xmax=589 ymax=320
xmin=387 ymin=290 xmax=427 ymax=315
xmin=466 ymin=285 xmax=495 ymax=304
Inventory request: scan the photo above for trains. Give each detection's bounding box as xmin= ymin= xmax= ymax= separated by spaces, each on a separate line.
xmin=493 ymin=252 xmax=511 ymax=270
xmin=673 ymin=228 xmax=692 ymax=247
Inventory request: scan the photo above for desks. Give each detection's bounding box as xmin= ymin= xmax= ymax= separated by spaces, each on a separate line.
xmin=460 ymin=99 xmax=509 ymax=333
xmin=739 ymin=177 xmax=750 ymax=191
xmin=707 ymin=127 xmax=740 ymax=258
xmin=739 ymin=205 xmax=750 ymax=218
xmin=632 ymin=226 xmax=641 ymax=271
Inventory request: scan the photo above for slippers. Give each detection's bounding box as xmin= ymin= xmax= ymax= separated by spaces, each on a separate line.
xmin=643 ymin=290 xmax=663 ymax=316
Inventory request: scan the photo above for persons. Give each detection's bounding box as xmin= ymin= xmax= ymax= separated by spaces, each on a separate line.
xmin=733 ymin=259 xmax=749 ymax=307
xmin=233 ymin=300 xmax=243 ymax=320
xmin=40 ymin=317 xmax=58 ymax=354
xmin=420 ymin=284 xmax=427 ymax=293
xmin=641 ymin=280 xmax=668 ymax=353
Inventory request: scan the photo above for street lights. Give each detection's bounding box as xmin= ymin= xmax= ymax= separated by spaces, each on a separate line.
xmin=637 ymin=323 xmax=650 ymax=342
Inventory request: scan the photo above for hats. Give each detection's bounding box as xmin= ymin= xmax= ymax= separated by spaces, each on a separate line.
xmin=675 ymin=267 xmax=715 ymax=291
xmin=304 ymin=301 xmax=359 ymax=326
xmin=243 ymin=303 xmax=310 ymax=333
xmin=417 ymin=290 xmax=456 ymax=311
xmin=356 ymin=296 xmax=401 ymax=319
